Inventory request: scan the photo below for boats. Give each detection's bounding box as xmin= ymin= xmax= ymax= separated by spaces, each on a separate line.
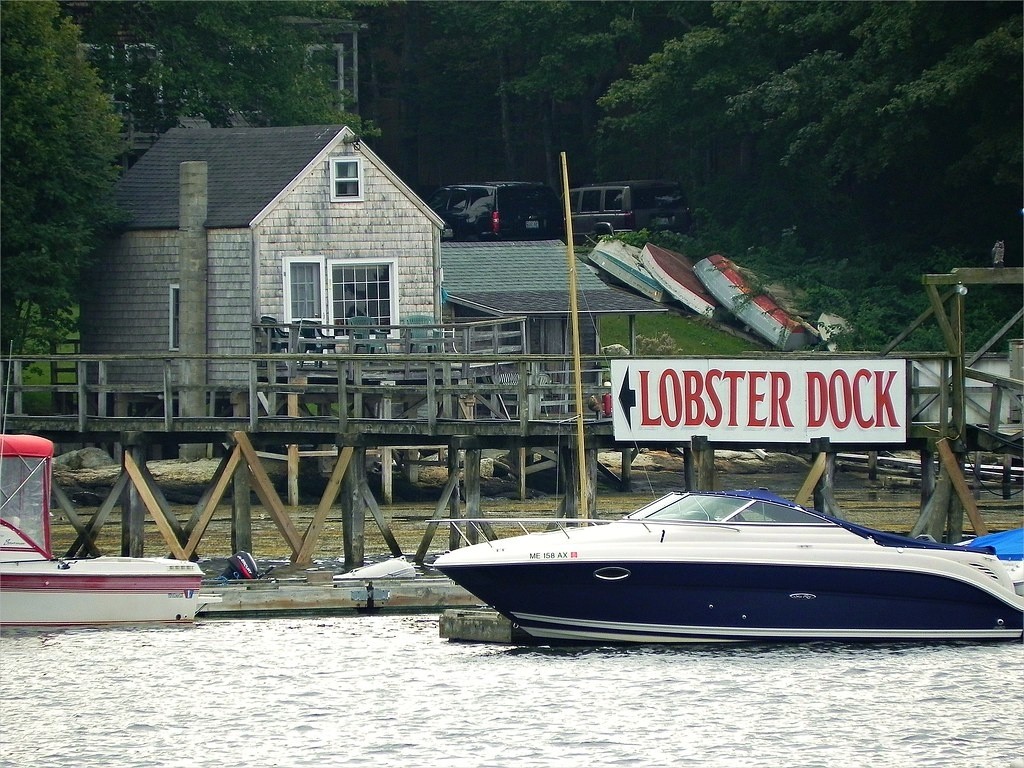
xmin=423 ymin=487 xmax=1024 ymax=648
xmin=586 ymin=234 xmax=681 ymax=304
xmin=0 ymin=436 xmax=204 ymax=626
xmin=693 ymin=255 xmax=821 ymax=351
xmin=950 ymin=529 xmax=1024 ymax=592
xmin=640 ymin=242 xmax=733 ymax=322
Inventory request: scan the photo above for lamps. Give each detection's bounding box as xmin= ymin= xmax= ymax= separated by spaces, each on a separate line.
xmin=345 ymin=135 xmax=362 ymax=150
xmin=955 ymin=283 xmax=968 ymax=295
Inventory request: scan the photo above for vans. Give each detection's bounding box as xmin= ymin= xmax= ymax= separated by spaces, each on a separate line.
xmin=423 ymin=181 xmax=565 ymax=242
xmin=570 ymin=180 xmax=695 ymax=241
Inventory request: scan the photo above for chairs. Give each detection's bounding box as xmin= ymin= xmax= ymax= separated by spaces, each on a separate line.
xmin=403 ymin=315 xmax=443 ymax=363
xmin=491 ymin=373 xmax=552 ymax=417
xmin=260 ymin=316 xmax=337 ymax=368
xmin=346 ymin=316 xmax=391 ymax=367
